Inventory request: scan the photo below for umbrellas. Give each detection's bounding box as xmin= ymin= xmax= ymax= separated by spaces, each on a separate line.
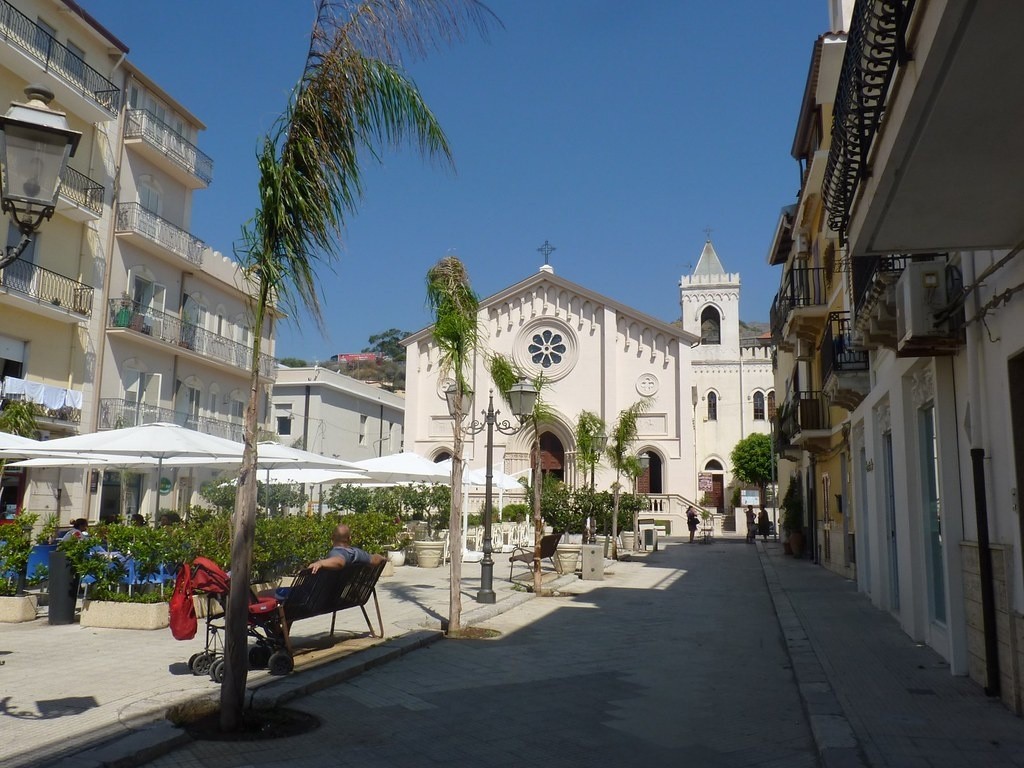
xmin=0 ymin=421 xmax=523 ymax=528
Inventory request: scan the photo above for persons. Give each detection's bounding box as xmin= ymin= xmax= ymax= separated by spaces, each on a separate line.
xmin=63 ymin=518 xmax=89 ymax=542
xmin=758 ymin=504 xmax=770 ymax=542
xmin=307 ymin=525 xmax=387 ymax=574
xmin=746 ymin=505 xmax=756 ymax=544
xmin=131 ymin=514 xmax=145 ymax=527
xmin=158 ymin=514 xmax=180 ymax=530
xmin=686 ymin=506 xmax=698 ymax=544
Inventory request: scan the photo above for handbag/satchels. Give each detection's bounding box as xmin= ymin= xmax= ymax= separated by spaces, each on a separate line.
xmin=693 ymin=518 xmax=699 ymax=524
xmin=169 ymin=564 xmax=197 ymax=640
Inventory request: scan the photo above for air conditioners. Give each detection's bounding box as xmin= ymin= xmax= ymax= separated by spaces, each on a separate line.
xmin=894 ymin=260 xmax=950 ymax=352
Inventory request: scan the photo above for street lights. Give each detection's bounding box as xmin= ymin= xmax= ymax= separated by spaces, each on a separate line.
xmin=579 ymin=427 xmax=609 ymax=545
xmin=620 ymin=452 xmax=650 ymax=546
xmin=444 ymin=372 xmax=540 ymax=605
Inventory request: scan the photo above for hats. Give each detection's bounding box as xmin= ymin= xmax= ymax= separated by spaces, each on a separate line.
xmin=131 ymin=514 xmax=143 ymax=521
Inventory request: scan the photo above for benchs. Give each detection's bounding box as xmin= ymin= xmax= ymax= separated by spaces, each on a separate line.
xmin=508 ymin=531 xmax=564 ymax=582
xmin=257 ymin=557 xmax=385 ymax=658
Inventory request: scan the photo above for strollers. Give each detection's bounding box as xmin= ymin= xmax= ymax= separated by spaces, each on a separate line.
xmin=186 ymin=554 xmax=296 ymax=684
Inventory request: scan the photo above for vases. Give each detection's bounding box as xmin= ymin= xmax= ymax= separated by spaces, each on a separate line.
xmin=129 ymin=312 xmax=145 ymax=333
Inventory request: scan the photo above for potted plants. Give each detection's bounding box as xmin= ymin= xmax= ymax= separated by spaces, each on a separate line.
xmin=179 ymin=309 xmax=193 ymax=349
xmin=0 ymin=479 xmax=451 ymax=631
xmin=519 ymin=474 xmax=649 ymax=575
xmin=776 ymin=469 xmax=806 ymax=557
